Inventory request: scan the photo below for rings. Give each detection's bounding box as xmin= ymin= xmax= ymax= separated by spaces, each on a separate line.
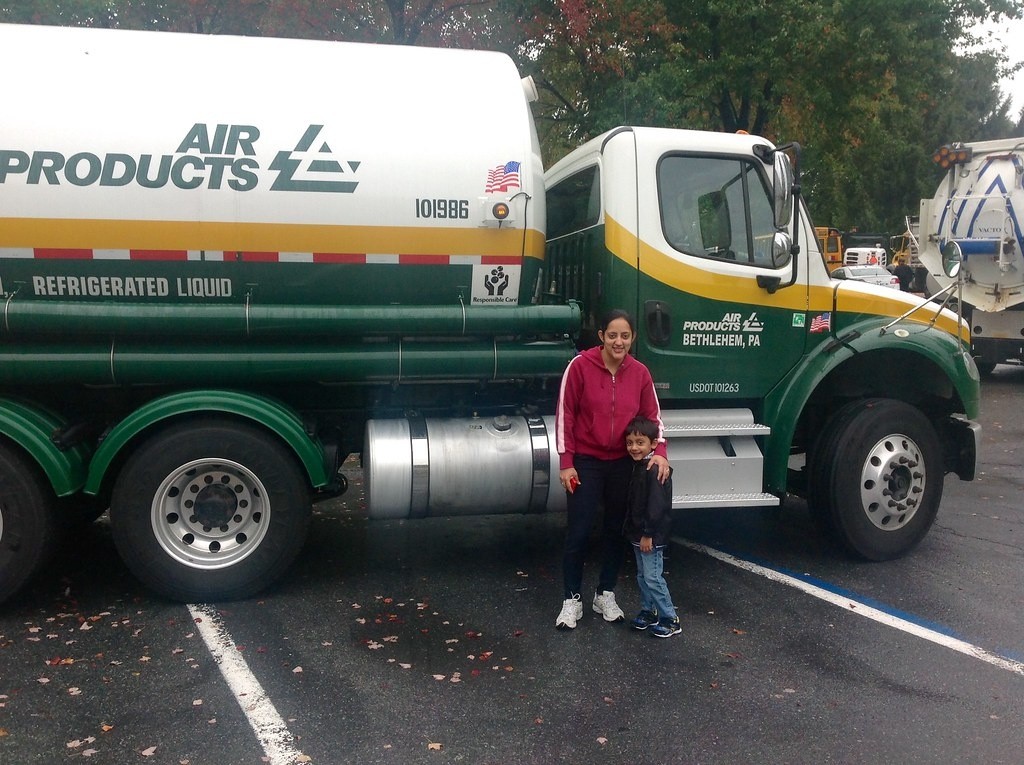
xmin=562 ymin=483 xmax=564 ymax=485
xmin=667 ymin=468 xmax=670 ymax=472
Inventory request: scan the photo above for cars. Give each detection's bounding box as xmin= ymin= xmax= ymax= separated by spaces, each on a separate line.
xmin=828 ymin=264 xmax=900 ymax=291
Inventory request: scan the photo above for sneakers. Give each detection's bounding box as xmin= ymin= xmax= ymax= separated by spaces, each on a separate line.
xmin=592 ymin=591 xmax=624 ymax=622
xmin=630 ymin=609 xmax=659 ymax=630
xmin=652 ymin=616 xmax=682 ymax=638
xmin=556 ymin=591 xmax=583 ymax=629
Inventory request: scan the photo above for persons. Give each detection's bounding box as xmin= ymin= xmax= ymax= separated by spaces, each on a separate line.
xmin=624 ymin=416 xmax=682 ymax=637
xmin=893 ymin=258 xmax=915 ymax=291
xmin=555 ymin=308 xmax=670 ymax=631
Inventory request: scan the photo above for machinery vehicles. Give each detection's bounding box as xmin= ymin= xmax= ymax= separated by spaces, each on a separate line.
xmin=0 ymin=24 xmax=985 ymax=603
xmin=751 ymin=227 xmax=857 ymax=275
xmin=906 ymin=135 xmax=1024 ymax=376
xmin=887 ymin=214 xmax=928 ymax=293
